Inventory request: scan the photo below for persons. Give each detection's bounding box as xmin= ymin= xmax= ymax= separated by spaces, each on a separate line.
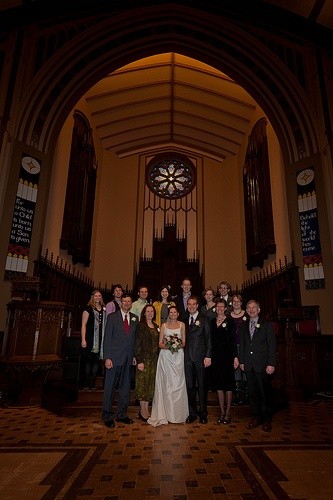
xmin=199 ymin=287 xmax=217 ymax=326
xmin=130 ymin=286 xmax=150 ymax=322
xmin=102 ymin=292 xmax=139 ymax=428
xmin=238 ymin=299 xmax=278 ymax=431
xmin=152 ymin=285 xmax=176 ymax=332
xmin=185 ymin=296 xmax=213 ymax=425
xmin=209 ymin=297 xmax=240 ymax=425
xmin=106 ymin=283 xmax=123 ymax=319
xmin=173 ymin=278 xmax=199 ymax=323
xmin=134 ymin=304 xmax=160 ymax=422
xmin=158 ymin=305 xmax=189 ymax=425
xmin=78 ymin=289 xmax=105 ymax=392
xmin=214 ymin=281 xmax=233 ymax=312
xmin=226 ymin=293 xmax=248 ymax=406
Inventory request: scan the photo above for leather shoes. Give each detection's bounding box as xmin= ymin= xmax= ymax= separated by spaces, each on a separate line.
xmin=186 ymin=415 xmax=197 ymax=423
xmin=105 ymin=420 xmax=115 ymax=428
xmin=117 ymin=417 xmax=133 ymax=423
xmin=199 ymin=415 xmax=208 ymax=424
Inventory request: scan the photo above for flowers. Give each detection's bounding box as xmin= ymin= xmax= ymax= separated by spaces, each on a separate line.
xmin=167 ymin=304 xmax=171 ymax=309
xmin=256 ymin=324 xmax=260 ymax=328
xmin=222 ymin=322 xmax=226 ymax=327
xmin=166 ymin=335 xmax=182 ymax=354
xmin=243 ymin=316 xmax=247 ymax=321
xmin=103 ymin=306 xmax=107 ymax=311
xmin=195 ymin=320 xmax=200 ymax=327
xmin=213 ymin=308 xmax=216 ymax=313
xmin=131 ymin=317 xmax=137 ymax=323
xmin=228 ymin=301 xmax=233 ymax=306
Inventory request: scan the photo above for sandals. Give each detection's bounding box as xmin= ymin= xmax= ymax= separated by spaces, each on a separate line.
xmin=218 ymin=416 xmax=224 ymax=423
xmin=223 ymin=415 xmax=231 ymax=424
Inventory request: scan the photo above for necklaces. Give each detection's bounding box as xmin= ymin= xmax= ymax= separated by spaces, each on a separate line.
xmin=233 ymin=311 xmax=242 ymax=316
xmin=216 ymin=316 xmax=226 ymax=327
xmin=206 ymin=303 xmax=214 ymax=308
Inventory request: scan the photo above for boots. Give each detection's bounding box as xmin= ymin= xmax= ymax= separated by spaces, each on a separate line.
xmin=243 ymin=381 xmax=250 ymax=405
xmin=232 ymin=380 xmax=242 ymax=405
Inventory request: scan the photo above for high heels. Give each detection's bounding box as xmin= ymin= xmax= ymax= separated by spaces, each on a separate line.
xmin=138 ymin=412 xmax=149 ymax=421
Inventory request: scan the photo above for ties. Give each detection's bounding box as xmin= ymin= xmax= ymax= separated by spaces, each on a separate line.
xmin=123 ymin=315 xmax=129 ymax=336
xmin=189 ymin=317 xmax=194 ymax=332
xmin=250 ymin=320 xmax=256 ymax=340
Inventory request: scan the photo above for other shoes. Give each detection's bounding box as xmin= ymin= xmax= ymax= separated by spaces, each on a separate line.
xmin=82 ymin=384 xmax=89 ymax=389
xmin=263 ymin=421 xmax=272 ymax=431
xmin=90 ymin=383 xmax=97 ymax=389
xmin=250 ymin=418 xmax=260 ymax=427
